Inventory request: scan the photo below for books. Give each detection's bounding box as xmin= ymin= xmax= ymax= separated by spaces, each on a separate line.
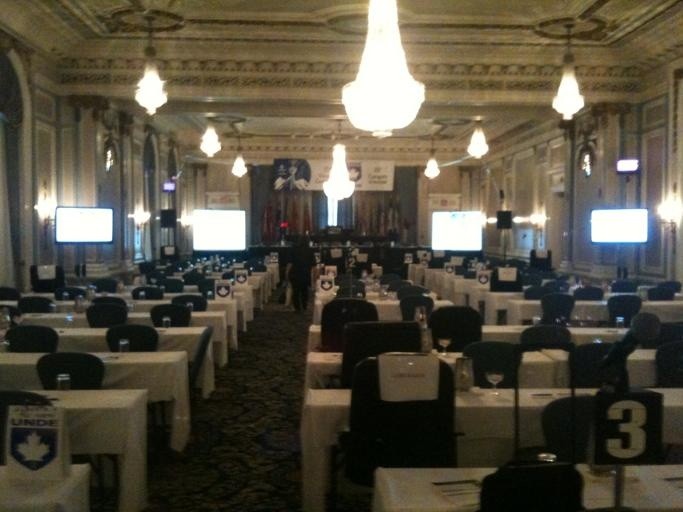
xmin=6 ymin=404 xmax=71 ymax=484
xmin=431 ymin=479 xmax=483 ymax=506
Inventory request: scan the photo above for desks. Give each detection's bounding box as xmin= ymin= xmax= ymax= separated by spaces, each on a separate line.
xmin=2 ymin=351 xmax=189 ymax=454
xmin=9 ymin=326 xmax=212 ymax=399
xmin=409 ymin=255 xmax=508 ymax=326
xmin=2 ymin=389 xmax=148 ymax=505
xmin=509 ymin=251 xmax=683 ymax=300
xmin=305 ymin=347 xmax=557 ymax=390
xmin=227 ymin=252 xmax=282 ymax=351
xmin=14 ymin=310 xmax=227 ymax=367
xmin=572 ymin=323 xmax=642 ymax=351
xmin=307 ymin=318 xmax=547 ymax=353
xmin=368 ymin=464 xmax=683 ymax=507
xmin=644 ymin=383 xmax=683 ymax=446
xmin=311 ymin=260 xmax=455 ymax=322
xmin=32 ymin=257 xmax=226 ymax=308
xmin=541 ymin=344 xmax=672 ymax=386
xmin=303 ymin=389 xmax=587 ymax=507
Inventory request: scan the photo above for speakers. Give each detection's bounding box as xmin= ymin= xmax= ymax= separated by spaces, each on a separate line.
xmin=160 ymin=208 xmax=177 ymax=228
xmin=496 ymin=210 xmax=513 ymax=229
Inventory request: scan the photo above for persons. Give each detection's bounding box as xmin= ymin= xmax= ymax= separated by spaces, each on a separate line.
xmin=286 ymin=245 xmax=309 ymax=313
xmin=285 ymin=281 xmax=293 ymax=309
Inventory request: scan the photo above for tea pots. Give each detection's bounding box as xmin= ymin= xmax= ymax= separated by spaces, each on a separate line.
xmin=86 ymin=285 xmax=95 ymax=301
xmin=456 ymin=357 xmax=474 ymax=392
xmin=75 ymin=295 xmax=85 ymax=314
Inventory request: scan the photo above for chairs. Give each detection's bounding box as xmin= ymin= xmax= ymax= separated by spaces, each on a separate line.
xmin=479 ymin=462 xmax=585 ymax=508
xmin=34 ymin=349 xmax=108 ymax=390
xmin=9 ymin=325 xmax=58 ymax=354
xmin=150 ymin=304 xmax=191 ymax=327
xmin=430 ymin=306 xmax=482 ymax=353
xmin=575 ymin=342 xmax=629 ymax=390
xmin=0 ymin=389 xmax=57 ymax=464
xmin=608 ymin=294 xmax=644 ymax=325
xmin=104 ymin=325 xmax=160 ymax=351
xmin=519 ymin=326 xmax=573 ymax=350
xmin=465 ymin=340 xmax=522 ymax=391
xmin=86 ymin=304 xmax=128 ymax=327
xmin=345 ymin=355 xmax=458 ymax=489
xmin=655 ymin=342 xmax=683 ymax=389
xmin=342 ymin=338 xmax=388 ymax=381
xmin=541 ymin=393 xmax=618 ymax=464
xmin=317 ymin=297 xmax=380 ymax=350
xmin=541 ymin=293 xmax=573 ymax=326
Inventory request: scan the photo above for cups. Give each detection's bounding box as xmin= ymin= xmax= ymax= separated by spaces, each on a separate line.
xmin=163 ymin=317 xmax=171 ymax=331
xmin=66 ymin=316 xmax=74 ymax=329
xmin=49 ymin=304 xmax=56 ymax=316
xmin=119 ymin=340 xmax=130 ymax=359
xmin=439 ymin=338 xmax=451 ymax=358
xmin=139 ymin=291 xmax=146 ymax=302
xmin=485 ymin=364 xmax=504 ymax=396
xmin=616 ymin=316 xmax=624 ymax=334
xmin=187 ymin=302 xmax=193 ymax=312
xmin=58 ymin=373 xmax=71 ymax=400
xmin=207 ymin=291 xmax=213 ymax=302
xmin=62 ymin=292 xmax=70 ymax=304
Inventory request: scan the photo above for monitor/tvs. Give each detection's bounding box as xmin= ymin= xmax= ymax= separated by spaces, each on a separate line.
xmin=54 ymin=206 xmax=115 ymax=243
xmin=589 ymin=207 xmax=650 ymax=245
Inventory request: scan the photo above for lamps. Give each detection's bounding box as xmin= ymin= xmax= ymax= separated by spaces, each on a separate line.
xmin=552 ymin=26 xmax=584 ymax=121
xmin=232 ymin=136 xmax=248 ymax=178
xmin=466 ymin=118 xmax=490 ymax=161
xmin=134 ymin=17 xmax=168 ymax=116
xmin=201 ymin=124 xmax=222 ymax=160
xmin=323 ymin=143 xmax=356 ymax=201
xmin=424 ymin=142 xmax=442 ymax=181
xmin=341 ymin=0 xmax=425 ymax=141
